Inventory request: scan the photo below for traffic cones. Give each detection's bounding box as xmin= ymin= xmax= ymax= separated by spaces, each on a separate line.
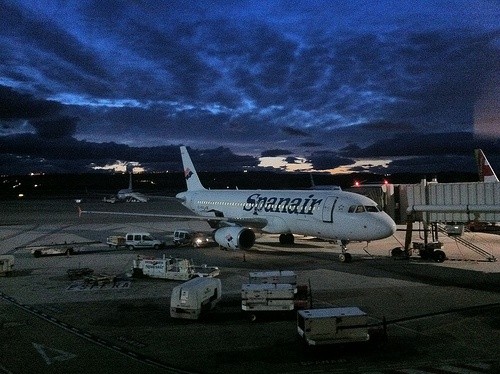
xmin=242 ymin=252 xmax=247 ymax=263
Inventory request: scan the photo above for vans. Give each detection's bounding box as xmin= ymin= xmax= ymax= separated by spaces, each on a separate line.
xmin=126 ymin=233 xmax=164 ymax=250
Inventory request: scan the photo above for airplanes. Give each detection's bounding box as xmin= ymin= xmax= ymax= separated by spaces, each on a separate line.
xmin=102 ymin=175 xmax=172 ymax=203
xmin=78 ymin=145 xmax=396 ymax=262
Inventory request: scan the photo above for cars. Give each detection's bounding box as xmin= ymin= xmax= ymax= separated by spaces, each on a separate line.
xmin=174 ymin=230 xmax=189 ymax=239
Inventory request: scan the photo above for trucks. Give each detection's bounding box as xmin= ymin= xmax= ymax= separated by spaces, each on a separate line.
xmin=171 ymin=277 xmax=222 ymax=320
xmin=240 ymin=284 xmax=309 ymax=322
